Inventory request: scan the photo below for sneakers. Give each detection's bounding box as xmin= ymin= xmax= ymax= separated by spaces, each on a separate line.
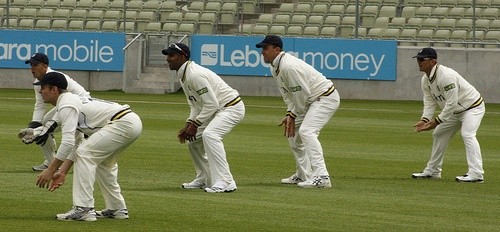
xmin=455 ymin=174 xmax=484 ymax=183
xmin=55 ymin=205 xmax=97 ymax=222
xmin=281 ymin=173 xmax=304 ymax=184
xmin=298 ymin=175 xmax=331 ymax=189
xmin=412 ymin=172 xmax=442 ymax=180
xmin=32 ymin=163 xmax=48 ymax=172
xmin=181 ymin=179 xmax=206 ymax=189
xmin=95 ymin=208 xmax=130 ymax=219
xmin=204 ymin=182 xmax=238 ymax=193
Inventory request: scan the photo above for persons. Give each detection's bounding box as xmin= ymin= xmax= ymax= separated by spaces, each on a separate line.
xmin=25 ymin=53 xmax=92 ymax=172
xmin=412 ymin=48 xmax=487 ymax=184
xmin=257 ymin=34 xmax=340 ymax=189
xmin=161 ymin=43 xmax=246 ymax=193
xmin=21 ymin=70 xmax=143 ymax=222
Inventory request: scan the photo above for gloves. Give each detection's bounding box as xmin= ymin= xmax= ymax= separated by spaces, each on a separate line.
xmin=18 ymin=121 xmax=42 ymax=144
xmin=32 ymin=120 xmax=58 ymax=146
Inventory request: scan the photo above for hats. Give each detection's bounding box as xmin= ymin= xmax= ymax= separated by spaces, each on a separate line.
xmin=256 ymin=35 xmax=283 ymax=50
xmin=412 ymin=48 xmax=437 ymax=59
xmin=25 ymin=53 xmax=49 ymax=65
xmin=33 ymin=72 xmax=68 ymax=90
xmin=162 ymin=43 xmax=191 ymax=60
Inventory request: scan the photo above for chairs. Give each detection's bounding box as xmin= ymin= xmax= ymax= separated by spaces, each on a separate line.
xmin=1 ymin=0 xmax=500 ymax=48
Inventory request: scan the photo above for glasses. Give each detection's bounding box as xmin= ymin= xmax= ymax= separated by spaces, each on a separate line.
xmin=417 ymin=57 xmax=432 ymax=62
xmin=168 ymin=42 xmax=189 ymax=57
